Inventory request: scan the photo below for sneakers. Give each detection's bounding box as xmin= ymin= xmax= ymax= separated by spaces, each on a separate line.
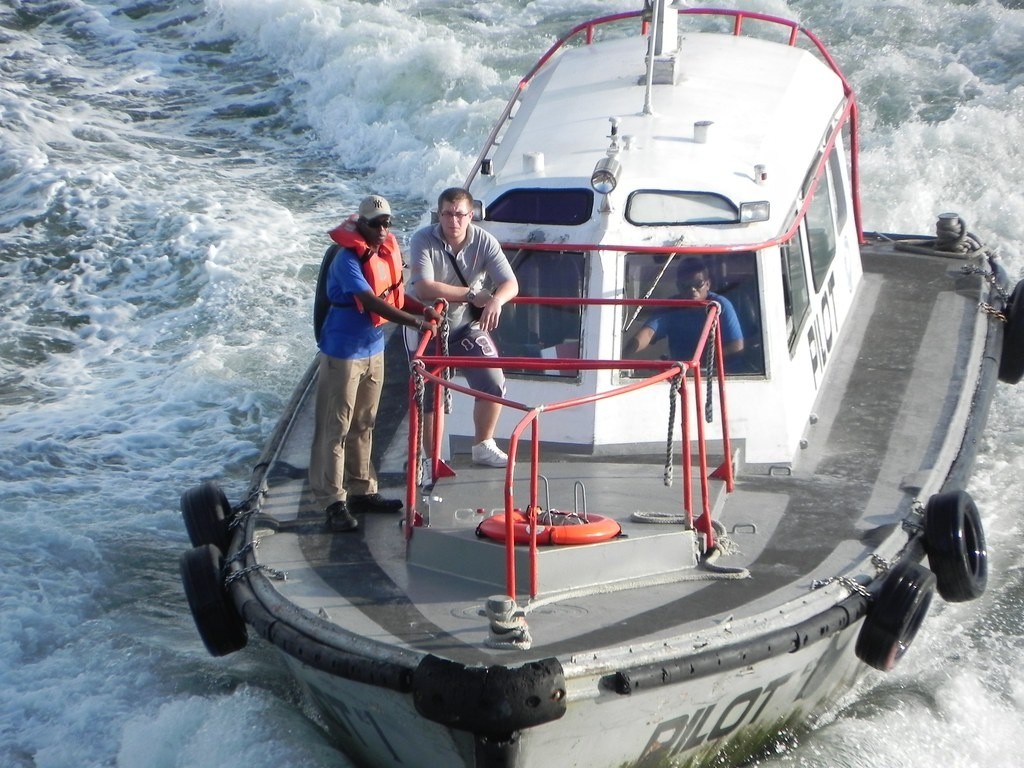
xmin=347 ymin=494 xmax=403 ymax=513
xmin=422 ymin=458 xmax=445 ymax=489
xmin=472 ymin=438 xmax=515 ymax=467
xmin=326 ymin=501 xmax=358 ymax=531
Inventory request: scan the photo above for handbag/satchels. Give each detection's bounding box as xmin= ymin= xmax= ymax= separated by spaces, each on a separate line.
xmin=471 ymin=304 xmax=485 ymax=320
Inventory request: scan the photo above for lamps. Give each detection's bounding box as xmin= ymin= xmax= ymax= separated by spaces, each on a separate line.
xmin=591 ymin=157 xmax=622 ymax=194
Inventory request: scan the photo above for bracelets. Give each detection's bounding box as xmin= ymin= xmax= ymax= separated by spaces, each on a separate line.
xmin=423 ymin=306 xmax=434 ymax=316
xmin=417 ymin=319 xmax=424 ymax=332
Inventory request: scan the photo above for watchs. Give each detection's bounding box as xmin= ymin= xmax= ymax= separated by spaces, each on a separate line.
xmin=467 ymin=287 xmax=476 ymax=303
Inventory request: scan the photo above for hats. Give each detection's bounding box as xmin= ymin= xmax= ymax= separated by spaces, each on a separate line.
xmin=359 ymin=195 xmax=395 ymax=220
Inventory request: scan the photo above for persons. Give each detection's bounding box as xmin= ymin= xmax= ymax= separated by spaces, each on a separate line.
xmin=622 ymin=258 xmax=745 ymax=382
xmin=403 ymin=187 xmax=519 ymax=488
xmin=315 ymin=195 xmax=444 ymax=536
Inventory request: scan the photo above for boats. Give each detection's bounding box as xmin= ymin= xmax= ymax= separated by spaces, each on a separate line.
xmin=179 ymin=0 xmax=1024 ymax=768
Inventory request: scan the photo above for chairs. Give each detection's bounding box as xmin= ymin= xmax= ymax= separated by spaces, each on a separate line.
xmin=713 ymin=277 xmax=761 ymax=372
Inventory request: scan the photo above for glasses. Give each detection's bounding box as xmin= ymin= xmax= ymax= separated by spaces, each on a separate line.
xmin=362 ymin=219 xmax=392 ymax=228
xmin=678 ymin=280 xmax=708 ymax=289
xmin=440 ymin=210 xmax=470 ymax=218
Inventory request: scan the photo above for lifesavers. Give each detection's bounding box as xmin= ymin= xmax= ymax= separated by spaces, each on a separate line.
xmin=176 ymin=476 xmax=234 ymax=549
xmin=995 ymin=278 xmax=1024 ymax=386
xmin=852 ymin=557 xmax=942 ymax=676
xmin=920 ymin=485 xmax=992 ymax=604
xmin=475 ymin=506 xmax=622 ymax=546
xmin=175 ymin=538 xmax=253 ymax=659
